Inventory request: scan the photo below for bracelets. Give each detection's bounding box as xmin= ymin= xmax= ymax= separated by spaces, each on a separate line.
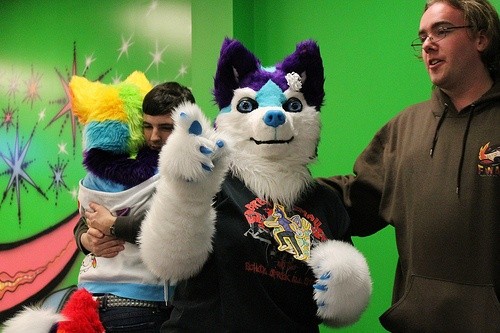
xmin=110 ymin=220 xmax=117 ymax=236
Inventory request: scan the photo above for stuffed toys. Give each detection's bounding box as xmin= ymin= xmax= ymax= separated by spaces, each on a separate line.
xmin=62 ymin=71 xmax=178 ymax=333
xmin=139 ymin=36 xmax=372 ymax=333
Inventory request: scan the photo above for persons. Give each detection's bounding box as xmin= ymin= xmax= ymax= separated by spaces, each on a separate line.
xmin=74 ymin=83 xmax=199 ymax=258
xmin=313 ymin=0 xmax=500 ymax=333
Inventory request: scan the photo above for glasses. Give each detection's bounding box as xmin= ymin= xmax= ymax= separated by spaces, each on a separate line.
xmin=411 ymin=25 xmax=473 ymax=51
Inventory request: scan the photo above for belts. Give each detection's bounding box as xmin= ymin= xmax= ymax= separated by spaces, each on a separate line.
xmin=99 ymin=294 xmax=160 ymax=307
